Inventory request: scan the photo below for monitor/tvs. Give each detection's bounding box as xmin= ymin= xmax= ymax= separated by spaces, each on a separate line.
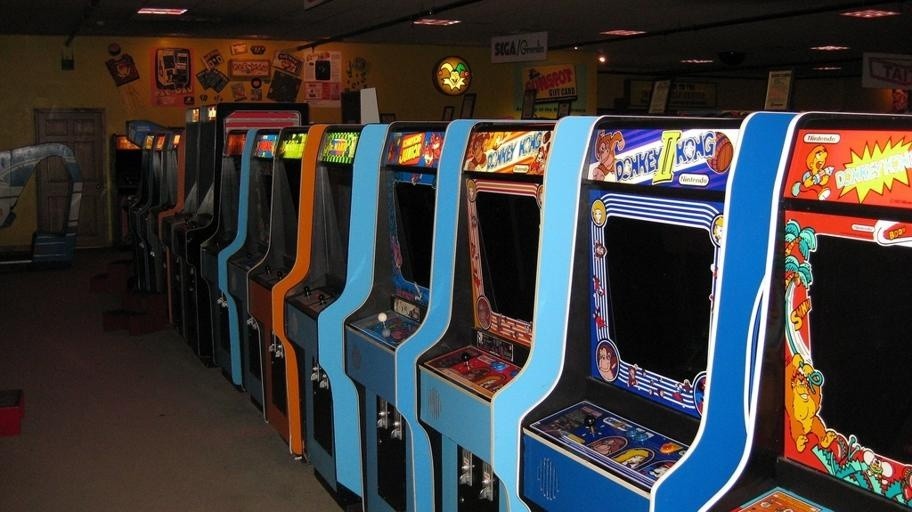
xmin=477 ymin=194 xmax=540 ymax=322
xmin=319 ymin=163 xmax=353 ymax=296
xmin=811 ymin=237 xmax=908 ymax=463
xmin=603 ymin=216 xmax=715 ymax=387
xmin=394 ymin=182 xmax=435 ymax=288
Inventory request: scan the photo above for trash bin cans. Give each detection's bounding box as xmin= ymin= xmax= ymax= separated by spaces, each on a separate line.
xmin=0 ymin=389 xmax=25 ymax=437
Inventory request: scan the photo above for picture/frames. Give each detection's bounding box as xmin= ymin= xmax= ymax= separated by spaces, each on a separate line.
xmin=379 ymin=113 xmax=396 ymax=124
xmin=557 ymin=101 xmax=571 ymax=120
xmin=763 ymin=66 xmax=794 ymax=112
xmin=460 ymin=93 xmax=476 ymax=119
xmin=647 ymin=78 xmax=673 ymax=115
xmin=442 ymin=106 xmax=455 ymax=121
xmin=521 ymin=90 xmax=537 ymax=119
